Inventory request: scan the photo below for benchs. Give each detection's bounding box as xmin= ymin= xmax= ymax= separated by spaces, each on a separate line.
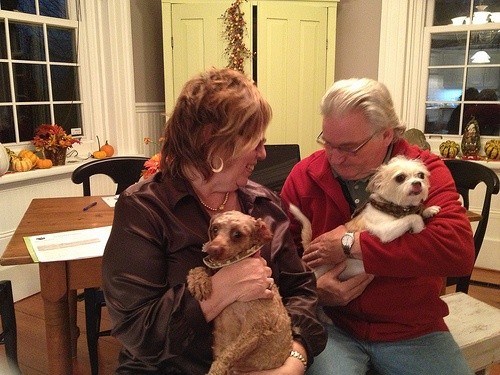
xmin=439 ymin=291 xmax=500 ymax=375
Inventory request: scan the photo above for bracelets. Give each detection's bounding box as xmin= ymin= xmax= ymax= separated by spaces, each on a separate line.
xmin=290 ymin=351 xmax=307 ymax=373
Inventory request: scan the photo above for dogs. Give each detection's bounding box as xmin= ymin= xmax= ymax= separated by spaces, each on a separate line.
xmin=288 ymin=155 xmax=441 ymax=279
xmin=187 ymin=210 xmax=292 ymax=375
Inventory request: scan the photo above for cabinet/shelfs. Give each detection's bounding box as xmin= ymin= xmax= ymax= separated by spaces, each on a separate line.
xmin=161 ymin=0 xmax=339 ymax=163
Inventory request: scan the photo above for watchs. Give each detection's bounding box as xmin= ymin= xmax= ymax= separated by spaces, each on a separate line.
xmin=341 ymin=232 xmax=355 ymax=257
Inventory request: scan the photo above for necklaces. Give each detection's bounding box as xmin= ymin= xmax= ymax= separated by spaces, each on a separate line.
xmin=197 ymin=192 xmax=229 ymax=211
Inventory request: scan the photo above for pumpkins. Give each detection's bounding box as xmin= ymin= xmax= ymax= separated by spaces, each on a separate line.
xmin=93 ymin=136 xmax=114 ymax=159
xmin=0 ymin=142 xmax=52 ymax=176
xmin=439 ymin=141 xmax=460 ymax=159
xmin=484 ymin=138 xmax=500 ymax=160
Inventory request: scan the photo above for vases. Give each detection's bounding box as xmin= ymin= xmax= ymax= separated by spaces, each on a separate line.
xmin=44 ymin=147 xmax=68 ymax=165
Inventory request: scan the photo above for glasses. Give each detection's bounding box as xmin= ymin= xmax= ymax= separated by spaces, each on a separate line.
xmin=239 ymin=130 xmax=266 ymax=151
xmin=316 ymin=128 xmax=382 ymax=157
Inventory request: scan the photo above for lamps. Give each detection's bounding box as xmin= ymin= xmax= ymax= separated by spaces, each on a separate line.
xmin=451 ymin=0 xmax=500 ymax=63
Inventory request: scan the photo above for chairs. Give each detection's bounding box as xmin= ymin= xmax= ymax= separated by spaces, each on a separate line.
xmin=442 ymin=160 xmax=500 ymax=293
xmin=0 ymin=280 xmax=23 ymax=375
xmin=72 ymin=156 xmax=151 ymax=375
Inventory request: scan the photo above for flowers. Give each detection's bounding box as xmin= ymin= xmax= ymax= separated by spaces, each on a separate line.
xmin=32 ymin=123 xmax=81 ymax=153
xmin=141 ymin=136 xmax=164 ymax=180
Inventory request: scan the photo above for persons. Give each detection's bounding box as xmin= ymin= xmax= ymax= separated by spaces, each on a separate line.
xmin=279 ymin=77 xmax=475 ymax=375
xmin=448 ymin=87 xmax=500 ymax=135
xmin=99 ymin=69 xmax=329 ymax=375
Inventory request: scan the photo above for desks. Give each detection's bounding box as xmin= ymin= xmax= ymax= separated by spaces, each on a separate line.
xmin=0 ymin=195 xmax=121 ymax=375
xmin=457 ymin=161 xmax=500 ymax=286
